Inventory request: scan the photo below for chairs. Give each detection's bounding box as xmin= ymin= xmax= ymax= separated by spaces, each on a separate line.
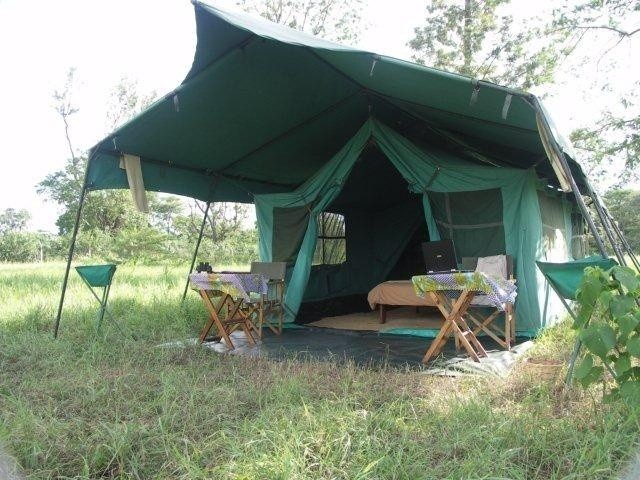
xmin=451 ymin=253 xmax=517 ymax=351
xmin=242 ymin=261 xmax=286 ymax=340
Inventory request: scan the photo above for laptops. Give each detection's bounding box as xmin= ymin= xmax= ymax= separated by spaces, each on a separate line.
xmin=421 ymin=239 xmax=475 ymax=274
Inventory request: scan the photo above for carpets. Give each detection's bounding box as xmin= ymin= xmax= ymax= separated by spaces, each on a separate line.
xmin=301 ymin=307 xmax=444 ymax=332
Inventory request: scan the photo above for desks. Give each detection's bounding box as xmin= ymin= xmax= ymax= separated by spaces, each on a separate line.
xmin=412 ymin=270 xmax=519 ymax=368
xmin=189 ymin=272 xmax=261 ymax=353
xmin=74 ymin=263 xmax=131 ymax=339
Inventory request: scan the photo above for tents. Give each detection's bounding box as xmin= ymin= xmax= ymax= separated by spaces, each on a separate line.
xmin=251 ymin=111 xmax=585 ymax=339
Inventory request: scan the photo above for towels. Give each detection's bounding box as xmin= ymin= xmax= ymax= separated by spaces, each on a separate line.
xmin=118 ymin=153 xmax=149 ymax=214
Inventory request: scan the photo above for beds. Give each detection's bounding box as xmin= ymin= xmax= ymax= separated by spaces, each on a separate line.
xmin=363 ymin=276 xmax=441 ymax=325
